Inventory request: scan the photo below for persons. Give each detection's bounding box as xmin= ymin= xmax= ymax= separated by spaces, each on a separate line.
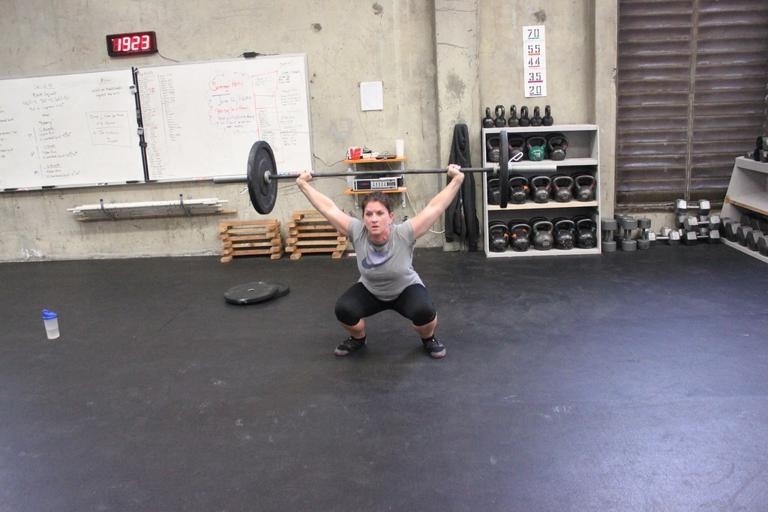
xmin=296 ymin=165 xmax=465 ymax=358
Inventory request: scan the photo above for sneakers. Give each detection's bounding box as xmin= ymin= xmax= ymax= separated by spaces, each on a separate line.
xmin=334 ymin=334 xmax=367 ymax=356
xmin=421 ymin=335 xmax=446 ymax=359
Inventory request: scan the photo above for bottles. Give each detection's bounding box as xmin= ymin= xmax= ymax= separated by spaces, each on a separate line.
xmin=346 ymin=167 xmax=354 ymax=191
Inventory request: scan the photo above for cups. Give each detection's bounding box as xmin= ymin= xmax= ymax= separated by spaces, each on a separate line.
xmin=348 ymin=146 xmax=360 ymax=161
xmin=396 ymin=141 xmax=404 ymax=160
xmin=43 ymin=309 xmax=60 ymax=341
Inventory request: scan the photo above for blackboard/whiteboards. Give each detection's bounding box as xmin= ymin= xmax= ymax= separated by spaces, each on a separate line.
xmin=0 ymin=53 xmax=314 ymax=192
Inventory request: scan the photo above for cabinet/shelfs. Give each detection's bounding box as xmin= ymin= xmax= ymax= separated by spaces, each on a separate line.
xmin=481 ymin=123 xmax=602 ymax=258
xmin=343 ymin=158 xmax=407 ymax=208
xmin=718 ymin=154 xmax=768 ymax=262
